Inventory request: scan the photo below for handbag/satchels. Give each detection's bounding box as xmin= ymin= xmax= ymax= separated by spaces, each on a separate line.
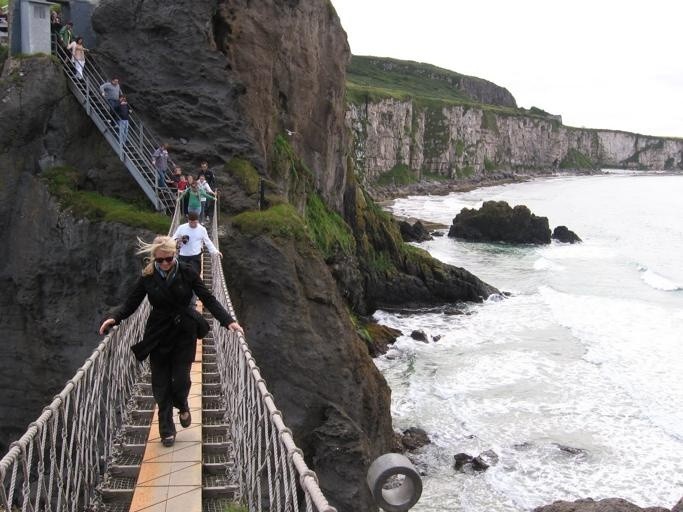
xmin=183 ymin=310 xmax=210 ymax=339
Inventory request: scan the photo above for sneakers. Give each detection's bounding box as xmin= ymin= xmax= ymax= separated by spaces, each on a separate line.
xmin=162 ymin=434 xmax=175 ymax=446
xmin=179 ymin=409 xmax=191 ymax=427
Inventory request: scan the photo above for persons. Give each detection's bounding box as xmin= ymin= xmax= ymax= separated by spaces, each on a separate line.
xmin=150 ymin=141 xmax=218 ymax=226
xmin=99 ymin=234 xmax=246 ymax=447
xmin=113 ymin=94 xmax=132 ymax=147
xmin=64 ymin=35 xmax=90 ymax=84
xmin=58 ymin=20 xmax=77 ymax=70
xmin=99 ymin=76 xmax=124 ymax=128
xmin=169 ymin=210 xmax=223 ymax=308
xmin=0 ymin=8 xmax=7 ymax=33
xmin=49 ymin=9 xmax=64 ymax=55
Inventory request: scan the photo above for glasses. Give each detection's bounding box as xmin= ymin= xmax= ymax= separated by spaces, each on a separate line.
xmin=155 ymin=255 xmax=174 ymax=263
